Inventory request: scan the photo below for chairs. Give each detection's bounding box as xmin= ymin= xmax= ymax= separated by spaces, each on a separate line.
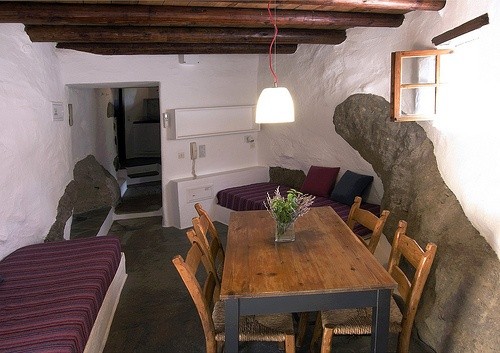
xmin=172 ymin=196 xmax=438 ymax=353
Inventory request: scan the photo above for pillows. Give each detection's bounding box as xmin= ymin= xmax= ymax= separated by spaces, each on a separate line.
xmin=270 ymin=167 xmax=308 ymax=188
xmin=302 ymin=165 xmax=341 ymax=198
xmin=332 ymin=170 xmax=373 ymax=206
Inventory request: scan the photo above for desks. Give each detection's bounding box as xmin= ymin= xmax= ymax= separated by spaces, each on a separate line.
xmin=219 ymin=206 xmax=398 ymax=353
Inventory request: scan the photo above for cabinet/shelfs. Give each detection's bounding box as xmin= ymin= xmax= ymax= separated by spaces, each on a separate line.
xmin=167 ymin=105 xmax=260 ymax=139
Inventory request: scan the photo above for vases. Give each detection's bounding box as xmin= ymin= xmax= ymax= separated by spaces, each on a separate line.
xmin=273 ymin=221 xmax=297 ymax=242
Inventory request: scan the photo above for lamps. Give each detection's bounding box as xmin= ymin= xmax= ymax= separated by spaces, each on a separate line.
xmin=255 ymin=0 xmax=295 ymax=124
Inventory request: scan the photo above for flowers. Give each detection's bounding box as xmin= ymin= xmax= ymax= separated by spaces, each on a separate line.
xmin=263 ymin=185 xmax=315 ymax=235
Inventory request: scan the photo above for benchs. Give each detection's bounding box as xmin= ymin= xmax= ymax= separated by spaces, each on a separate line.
xmin=0 ymin=236 xmax=129 ymax=353
xmin=216 ymin=181 xmax=392 ymax=264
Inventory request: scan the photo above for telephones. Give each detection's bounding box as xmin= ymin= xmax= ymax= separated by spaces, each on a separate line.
xmin=190 ymin=142 xmax=197 ymax=160
